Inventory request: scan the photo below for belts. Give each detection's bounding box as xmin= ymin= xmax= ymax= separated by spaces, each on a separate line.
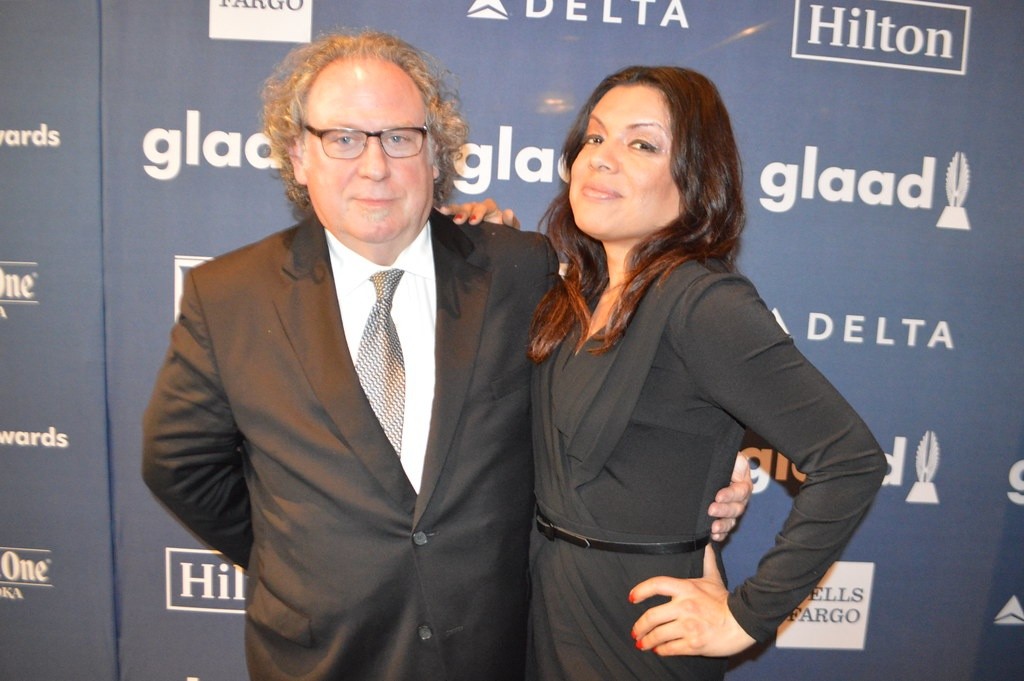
xmin=536 ymin=508 xmax=710 ymax=555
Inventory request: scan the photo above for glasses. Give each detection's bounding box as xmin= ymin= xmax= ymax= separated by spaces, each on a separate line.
xmin=302 ymin=122 xmax=428 ymax=160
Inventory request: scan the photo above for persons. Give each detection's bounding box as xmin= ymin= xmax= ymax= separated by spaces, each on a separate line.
xmin=142 ymin=33 xmax=752 ymax=681
xmin=438 ymin=65 xmax=887 ymax=681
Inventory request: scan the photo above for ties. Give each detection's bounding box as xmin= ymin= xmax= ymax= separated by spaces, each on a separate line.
xmin=356 ymin=269 xmax=406 ymax=459
